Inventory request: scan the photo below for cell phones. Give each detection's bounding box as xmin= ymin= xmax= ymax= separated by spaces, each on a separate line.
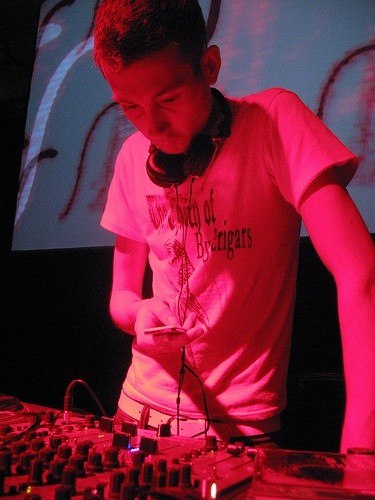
xmin=144 ymin=325 xmax=187 ymax=336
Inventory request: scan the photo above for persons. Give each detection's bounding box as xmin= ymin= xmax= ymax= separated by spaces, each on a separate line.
xmin=90 ymin=0 xmax=375 ymax=455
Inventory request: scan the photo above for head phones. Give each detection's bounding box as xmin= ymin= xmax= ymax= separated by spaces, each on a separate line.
xmin=145 ymin=87 xmax=232 ymax=188
